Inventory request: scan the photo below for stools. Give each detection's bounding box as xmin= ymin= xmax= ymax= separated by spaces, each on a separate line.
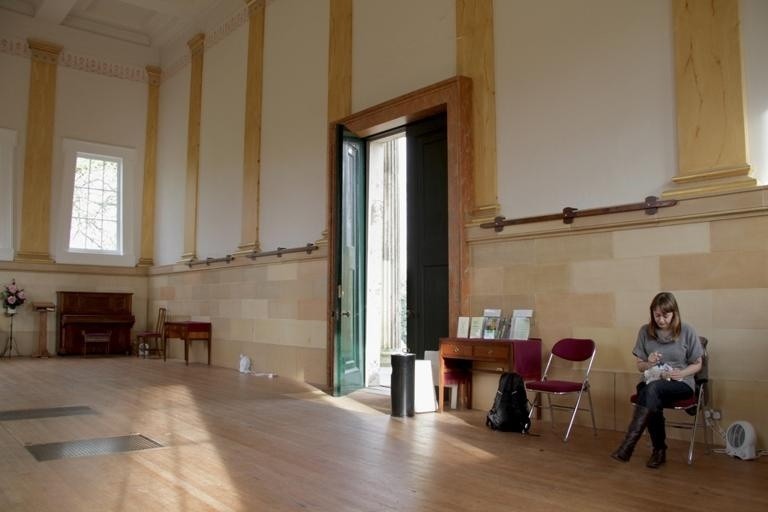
xmin=83 ymin=330 xmax=110 ymax=353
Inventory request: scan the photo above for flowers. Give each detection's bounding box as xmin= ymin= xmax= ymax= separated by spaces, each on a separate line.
xmin=1 ymin=280 xmax=26 ymax=308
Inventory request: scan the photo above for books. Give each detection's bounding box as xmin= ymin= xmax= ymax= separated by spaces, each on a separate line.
xmin=456 ymin=308 xmax=534 ymax=340
xmin=643 ymin=363 xmax=672 ymax=387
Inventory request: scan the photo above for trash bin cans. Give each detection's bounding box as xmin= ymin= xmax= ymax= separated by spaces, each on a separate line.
xmin=390 ymin=352 xmax=415 ymax=417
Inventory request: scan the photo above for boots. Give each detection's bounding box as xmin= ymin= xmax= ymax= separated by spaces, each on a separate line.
xmin=647 ymin=414 xmax=667 ymax=468
xmin=611 ymin=405 xmax=652 ymax=462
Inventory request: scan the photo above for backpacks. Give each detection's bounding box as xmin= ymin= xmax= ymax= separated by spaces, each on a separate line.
xmin=487 ymin=371 xmax=530 ymax=434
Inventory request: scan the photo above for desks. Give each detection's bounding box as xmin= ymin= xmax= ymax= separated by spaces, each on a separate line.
xmin=163 ymin=321 xmax=212 ymax=365
xmin=437 ymin=337 xmax=542 ymax=422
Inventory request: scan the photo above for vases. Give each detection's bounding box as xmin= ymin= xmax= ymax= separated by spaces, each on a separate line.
xmin=5 ymin=304 xmax=16 ymax=314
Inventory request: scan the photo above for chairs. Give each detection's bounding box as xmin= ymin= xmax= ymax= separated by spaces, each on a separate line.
xmin=630 ymin=335 xmax=709 ymax=463
xmin=137 ymin=308 xmax=168 ymax=358
xmin=522 ymin=336 xmax=598 ymax=441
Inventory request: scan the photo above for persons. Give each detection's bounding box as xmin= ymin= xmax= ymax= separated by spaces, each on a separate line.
xmin=609 ymin=292 xmax=705 ymax=469
